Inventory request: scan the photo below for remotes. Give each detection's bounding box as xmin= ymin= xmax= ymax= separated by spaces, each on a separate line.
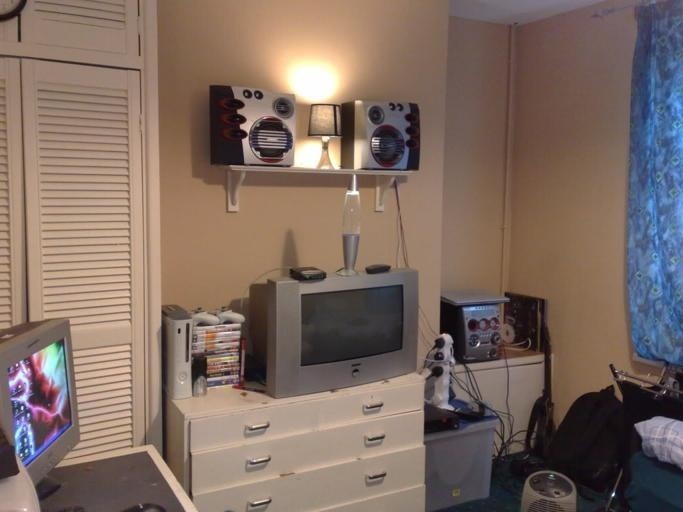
xmin=365 ymin=264 xmax=391 ymax=273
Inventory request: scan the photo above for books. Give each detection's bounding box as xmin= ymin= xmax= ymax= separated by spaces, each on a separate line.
xmin=192 ymin=320 xmax=247 ymax=392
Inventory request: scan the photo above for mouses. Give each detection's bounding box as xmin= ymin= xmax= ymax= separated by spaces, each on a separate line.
xmin=125 ymin=503 xmax=166 ymax=512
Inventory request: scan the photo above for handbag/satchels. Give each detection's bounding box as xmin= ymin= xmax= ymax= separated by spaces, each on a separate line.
xmin=546 ymin=382 xmax=639 ymax=492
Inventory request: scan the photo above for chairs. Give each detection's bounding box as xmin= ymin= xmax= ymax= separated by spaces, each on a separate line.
xmin=538 ymin=392 xmax=623 ymax=512
xmin=624 ymin=451 xmax=683 ymax=512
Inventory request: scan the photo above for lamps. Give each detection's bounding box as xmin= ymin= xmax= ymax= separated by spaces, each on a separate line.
xmin=308 ymin=103 xmax=341 ymax=169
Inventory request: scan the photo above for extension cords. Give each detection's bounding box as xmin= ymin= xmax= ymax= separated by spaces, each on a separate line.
xmin=434 ymin=333 xmax=451 ymax=408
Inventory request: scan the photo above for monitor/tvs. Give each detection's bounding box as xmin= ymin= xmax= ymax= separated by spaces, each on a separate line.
xmin=250 ymin=270 xmax=418 ymax=399
xmin=0 ymin=318 xmax=81 ymax=501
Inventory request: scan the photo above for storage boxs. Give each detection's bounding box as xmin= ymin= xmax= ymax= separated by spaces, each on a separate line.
xmin=425 ymin=412 xmax=499 ymax=511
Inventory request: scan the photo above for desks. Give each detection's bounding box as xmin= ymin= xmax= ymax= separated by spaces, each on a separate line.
xmin=454 ymin=349 xmax=546 ymax=458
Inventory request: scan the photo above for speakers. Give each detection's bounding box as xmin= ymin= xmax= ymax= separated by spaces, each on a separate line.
xmin=210 ymin=84 xmax=298 ymax=168
xmin=520 ymin=469 xmax=577 ymax=512
xmin=340 ymin=99 xmax=420 ymax=170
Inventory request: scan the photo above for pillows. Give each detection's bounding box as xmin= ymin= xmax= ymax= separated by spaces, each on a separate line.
xmin=634 ymin=415 xmax=683 ymax=470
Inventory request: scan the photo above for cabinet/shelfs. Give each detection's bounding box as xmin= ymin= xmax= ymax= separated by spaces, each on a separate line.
xmin=191 ymin=383 xmax=426 ymax=512
